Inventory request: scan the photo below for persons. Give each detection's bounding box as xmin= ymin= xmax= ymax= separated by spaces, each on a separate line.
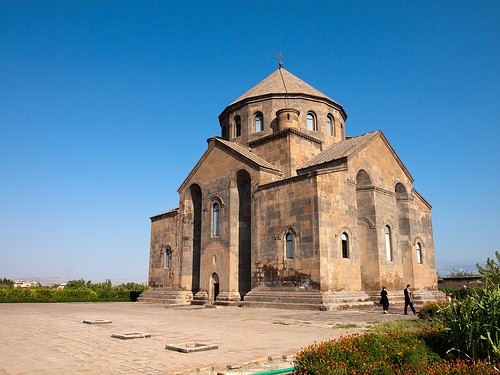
xmin=378 ymin=286 xmax=390 ymax=314
xmin=459 ymin=284 xmax=468 ymax=299
xmin=447 ymin=288 xmax=454 ymax=301
xmin=402 ymin=284 xmax=419 ymax=315
